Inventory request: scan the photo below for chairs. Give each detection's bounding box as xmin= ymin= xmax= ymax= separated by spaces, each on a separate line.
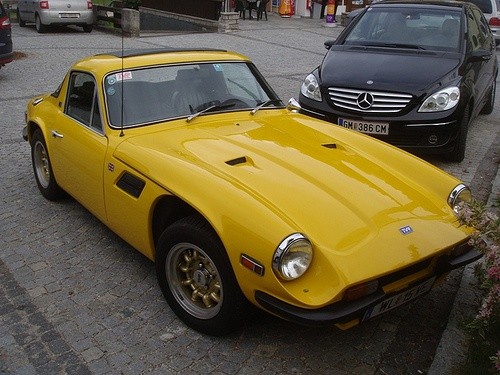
xmin=106 ymin=78 xmax=163 ymax=125
xmin=377 ymin=12 xmax=407 ymax=43
xmin=436 ymin=18 xmax=459 ymax=47
xmin=175 ymin=68 xmax=226 ymax=115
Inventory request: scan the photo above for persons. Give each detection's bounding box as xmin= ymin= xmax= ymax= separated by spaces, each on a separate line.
xmin=233 ymin=0 xmax=271 ymax=21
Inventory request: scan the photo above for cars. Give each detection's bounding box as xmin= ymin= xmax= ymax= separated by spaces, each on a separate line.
xmin=22 ymin=29 xmax=485 ymax=336
xmin=0 ymin=1 xmax=15 ymax=68
xmin=16 ymin=0 xmax=95 ymax=33
xmin=299 ymin=0 xmax=499 ymax=161
